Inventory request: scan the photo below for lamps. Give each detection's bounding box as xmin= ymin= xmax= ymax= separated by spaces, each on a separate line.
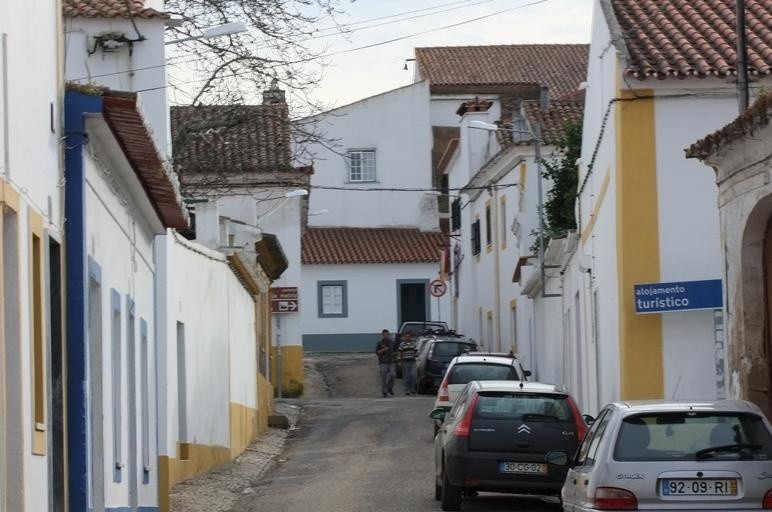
xmin=425 ymin=190 xmax=460 ymax=199
xmin=256 ymin=189 xmax=310 ymax=206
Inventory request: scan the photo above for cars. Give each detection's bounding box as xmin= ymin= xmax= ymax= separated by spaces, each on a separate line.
xmin=429 ymin=350 xmax=532 ymax=439
xmin=429 ymin=379 xmax=594 ymax=512
xmin=560 ymin=398 xmax=772 ymax=512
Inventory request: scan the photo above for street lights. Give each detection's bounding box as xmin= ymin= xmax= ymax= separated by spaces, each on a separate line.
xmin=307 ymin=209 xmax=328 ymax=216
xmin=423 ymin=190 xmax=460 ymax=197
xmin=256 ymin=189 xmax=308 ymax=227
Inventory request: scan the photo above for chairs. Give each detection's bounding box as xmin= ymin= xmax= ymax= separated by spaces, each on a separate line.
xmin=617 ymin=417 xmax=651 ymax=456
xmin=709 ymin=423 xmax=750 ymax=458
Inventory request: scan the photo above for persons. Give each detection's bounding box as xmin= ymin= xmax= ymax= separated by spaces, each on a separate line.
xmin=375 ymin=329 xmax=398 ymax=397
xmin=398 ymin=331 xmax=419 ymax=395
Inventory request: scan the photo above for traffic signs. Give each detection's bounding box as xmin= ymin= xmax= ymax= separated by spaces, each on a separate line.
xmin=271 ymin=286 xmax=299 ymax=313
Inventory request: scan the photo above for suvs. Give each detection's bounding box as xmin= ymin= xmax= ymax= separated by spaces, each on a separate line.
xmin=394 ymin=321 xmax=481 ymax=395
